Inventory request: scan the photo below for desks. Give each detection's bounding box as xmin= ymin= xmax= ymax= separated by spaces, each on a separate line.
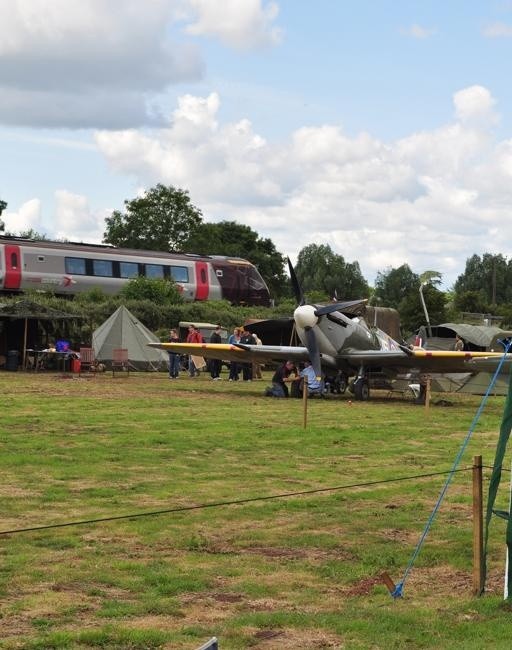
xmin=26 ymin=349 xmax=73 ymax=371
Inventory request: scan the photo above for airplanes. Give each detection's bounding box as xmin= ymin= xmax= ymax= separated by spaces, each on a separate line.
xmin=146 ymin=255 xmax=512 ymax=399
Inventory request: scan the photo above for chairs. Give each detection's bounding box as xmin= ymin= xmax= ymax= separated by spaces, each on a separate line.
xmin=111 ymin=349 xmax=128 ymax=376
xmin=78 ymin=348 xmax=95 ymax=377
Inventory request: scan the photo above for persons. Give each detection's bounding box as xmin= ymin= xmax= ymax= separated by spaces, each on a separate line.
xmin=208 ymin=325 xmax=221 ymax=381
xmin=262 ymin=359 xmax=299 ymax=401
xmin=166 ymin=328 xmax=181 ymax=379
xmin=185 ymin=325 xmax=202 ymax=378
xmin=297 ymin=362 xmax=328 ymax=400
xmin=455 ymin=334 xmax=464 ymax=351
xmin=239 ymin=331 xmax=255 ymax=383
xmin=251 ymin=333 xmax=263 ymax=380
xmin=227 ymin=327 xmax=241 ymax=383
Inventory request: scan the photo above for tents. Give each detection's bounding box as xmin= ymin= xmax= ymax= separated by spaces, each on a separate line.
xmin=84 ymin=304 xmax=169 ymax=373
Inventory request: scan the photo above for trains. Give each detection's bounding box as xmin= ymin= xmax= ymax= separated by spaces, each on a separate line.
xmin=0 ymin=236 xmax=271 ymax=307
